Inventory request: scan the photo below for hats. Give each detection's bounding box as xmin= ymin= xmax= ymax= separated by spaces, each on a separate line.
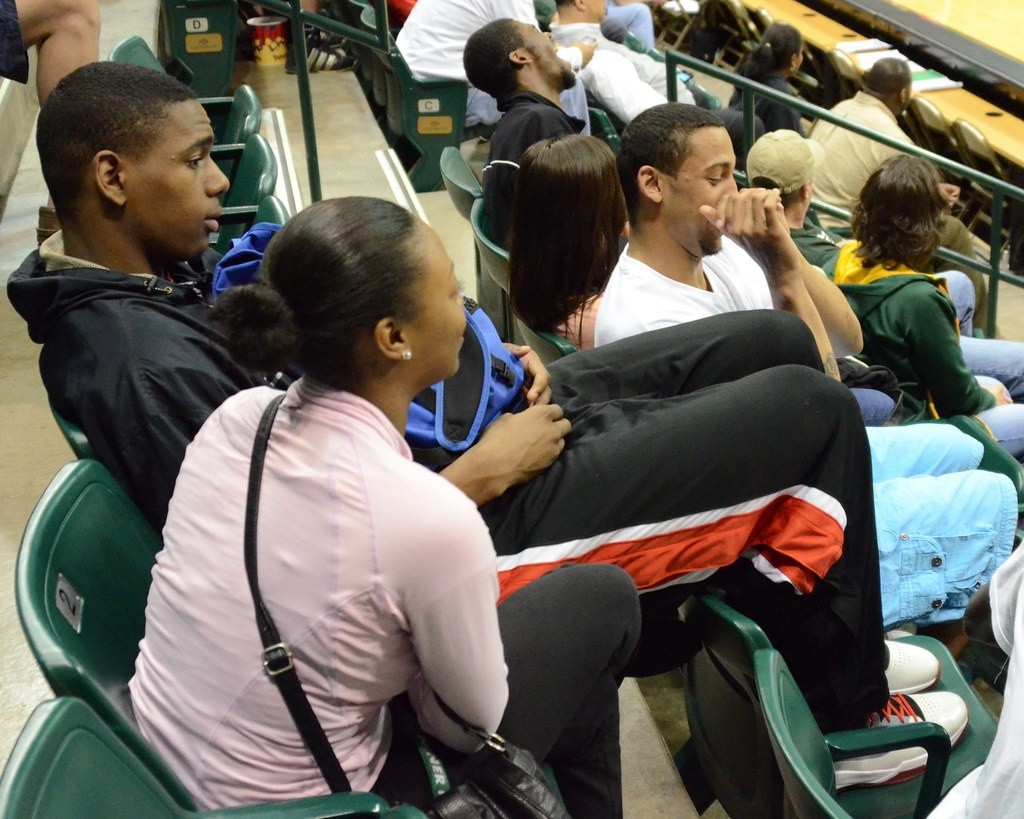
xmin=746 ymin=129 xmax=823 ymax=195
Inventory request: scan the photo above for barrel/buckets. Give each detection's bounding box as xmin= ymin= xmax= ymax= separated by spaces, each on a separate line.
xmin=247 ymin=16 xmax=286 ymax=66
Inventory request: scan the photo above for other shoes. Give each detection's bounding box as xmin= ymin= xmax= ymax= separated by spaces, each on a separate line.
xmin=36 ymin=206 xmax=61 ymax=244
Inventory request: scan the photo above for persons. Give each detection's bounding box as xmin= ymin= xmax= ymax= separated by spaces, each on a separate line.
xmin=0 ymin=0 xmax=102 ymax=248
xmin=593 ymin=103 xmax=1015 ymax=681
xmin=728 ymin=23 xmax=803 ymax=137
xmin=817 ymin=155 xmax=1024 ymax=457
xmin=128 ymin=197 xmax=640 ymax=819
xmin=463 ymin=19 xmax=585 ymax=244
xmin=508 ymin=135 xmax=895 ymax=428
xmin=546 ymin=0 xmax=763 ymax=166
xmin=397 ymin=0 xmax=599 ymax=135
xmin=809 ymin=58 xmax=987 ymax=333
xmin=926 ymin=537 xmax=1024 ymax=819
xmin=284 ymin=0 xmax=358 ymax=72
xmin=747 ymin=128 xmax=1024 ymax=400
xmin=605 ymin=0 xmax=663 ymax=53
xmin=5 ymin=64 xmax=968 ymax=788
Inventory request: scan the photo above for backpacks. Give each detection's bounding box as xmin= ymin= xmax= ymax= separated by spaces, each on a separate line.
xmin=210 ymin=222 xmax=537 ymax=471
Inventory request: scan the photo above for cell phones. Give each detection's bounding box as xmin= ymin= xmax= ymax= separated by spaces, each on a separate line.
xmin=678 ymin=70 xmax=693 ymax=83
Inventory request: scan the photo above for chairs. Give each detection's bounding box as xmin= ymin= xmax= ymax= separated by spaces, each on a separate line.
xmin=0 ymin=0 xmax=1024 ymax=819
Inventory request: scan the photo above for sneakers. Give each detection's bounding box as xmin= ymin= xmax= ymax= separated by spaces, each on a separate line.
xmin=831 ymin=690 xmax=970 ymax=795
xmin=283 ymin=26 xmax=358 ymax=74
xmin=884 ymin=638 xmax=940 ymax=695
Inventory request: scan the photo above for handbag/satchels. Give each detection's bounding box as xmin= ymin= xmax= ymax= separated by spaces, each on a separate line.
xmin=402 ymin=727 xmax=571 ymax=819
xmin=835 ymin=357 xmax=905 ymax=426
